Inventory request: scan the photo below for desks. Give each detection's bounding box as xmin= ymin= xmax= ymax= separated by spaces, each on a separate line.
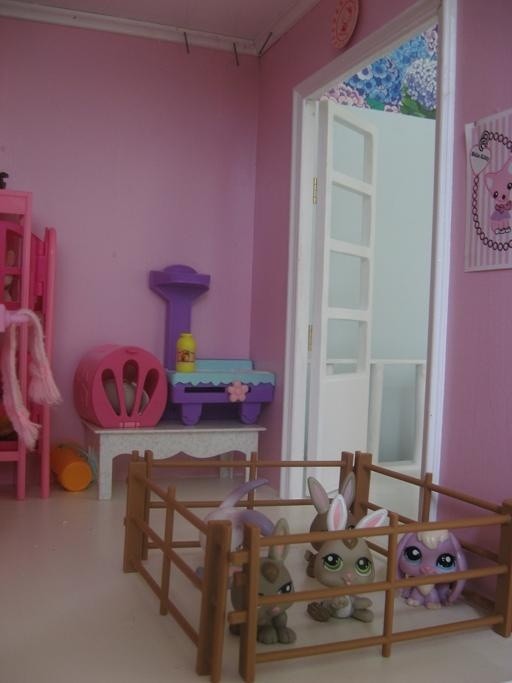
xmin=80 ymin=415 xmax=268 ymax=503
